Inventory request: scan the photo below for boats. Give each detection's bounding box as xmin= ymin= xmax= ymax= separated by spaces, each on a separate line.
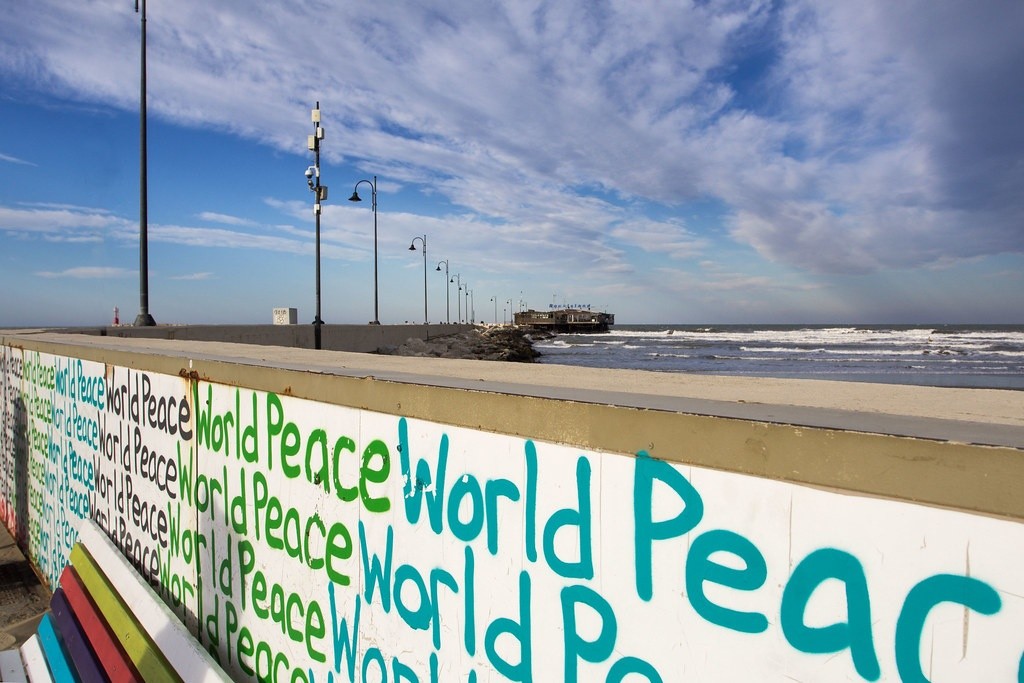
xmin=514 ymin=309 xmax=614 ymax=333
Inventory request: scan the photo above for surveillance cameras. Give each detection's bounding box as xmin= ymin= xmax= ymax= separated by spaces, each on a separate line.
xmin=304 ymin=166 xmax=314 ymax=178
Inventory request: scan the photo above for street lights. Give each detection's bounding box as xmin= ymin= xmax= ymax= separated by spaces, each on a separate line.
xmin=436 ymin=261 xmax=449 ymax=325
xmin=459 ymin=284 xmax=467 ymax=323
xmin=409 ymin=237 xmax=429 ymax=325
xmin=450 ymin=275 xmax=461 ymax=323
xmin=466 ymin=290 xmax=473 ymax=323
xmin=348 ymin=180 xmax=379 ymax=323
xmin=506 ymin=298 xmax=512 ymax=323
xmin=491 ymin=295 xmax=497 ymax=323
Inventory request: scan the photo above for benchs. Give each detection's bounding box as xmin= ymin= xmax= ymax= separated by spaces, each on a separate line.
xmin=0 ymin=517 xmax=235 ymax=683
